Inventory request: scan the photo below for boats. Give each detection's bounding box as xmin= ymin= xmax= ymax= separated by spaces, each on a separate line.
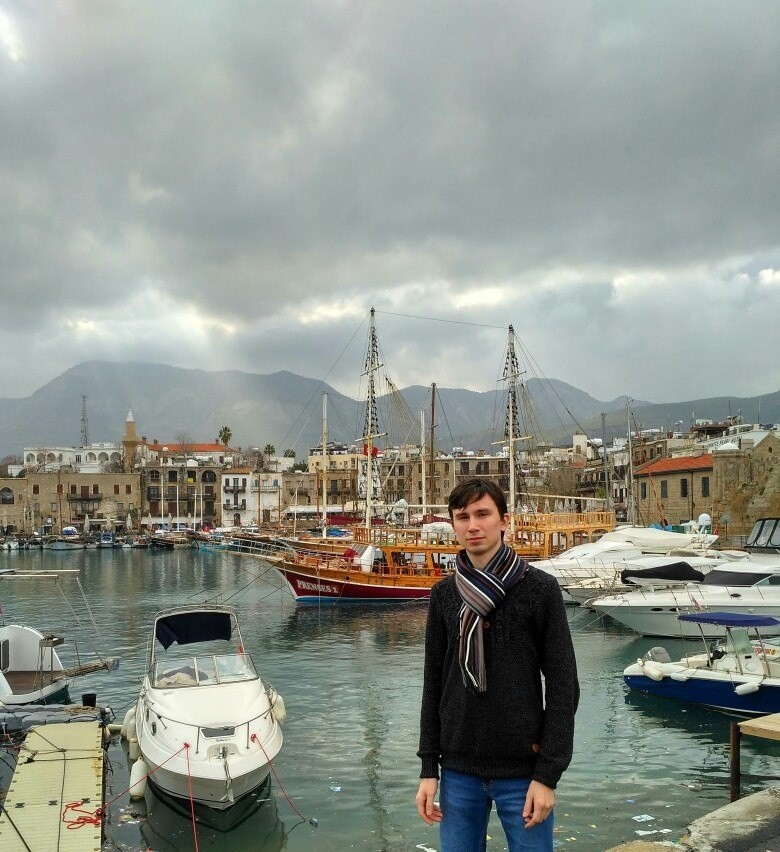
xmin=622 ymin=612 xmax=780 ymax=717
xmin=130 ymin=603 xmax=288 ymax=810
xmin=529 ymin=397 xmax=780 ymax=613
xmin=0 ymin=622 xmax=76 ymax=708
xmin=0 ymin=475 xmax=308 ymax=558
xmin=591 ymin=558 xmax=780 ymax=639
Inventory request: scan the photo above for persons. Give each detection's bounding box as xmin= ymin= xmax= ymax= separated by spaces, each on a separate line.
xmin=415 ymin=477 xmax=581 ymax=852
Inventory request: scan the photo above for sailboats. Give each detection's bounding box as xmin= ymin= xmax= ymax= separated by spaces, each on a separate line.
xmin=216 ymin=307 xmax=618 ymax=604
